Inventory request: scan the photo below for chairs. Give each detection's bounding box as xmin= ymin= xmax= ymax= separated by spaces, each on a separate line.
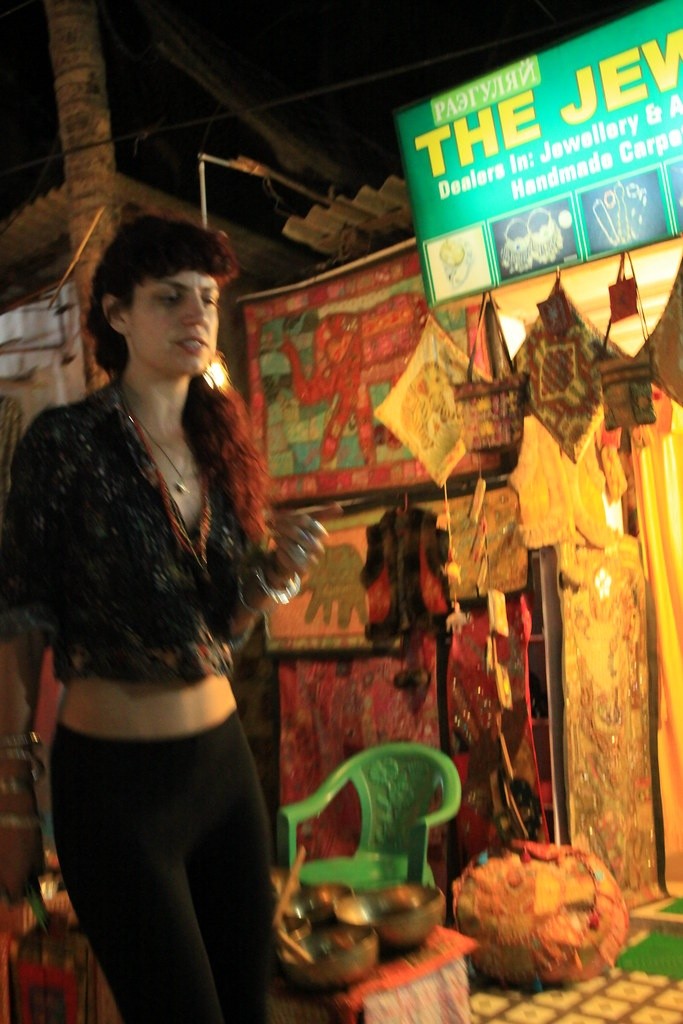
xmin=274 ymin=740 xmax=462 ymax=895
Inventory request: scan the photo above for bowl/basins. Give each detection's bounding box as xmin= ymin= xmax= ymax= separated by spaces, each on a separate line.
xmin=291 ymin=882 xmax=354 ymax=922
xmin=284 ymin=925 xmax=379 ymax=985
xmin=339 ymin=886 xmax=447 ymax=952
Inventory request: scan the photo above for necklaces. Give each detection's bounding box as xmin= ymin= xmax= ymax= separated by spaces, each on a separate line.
xmin=125 ymin=400 xmax=190 ymax=496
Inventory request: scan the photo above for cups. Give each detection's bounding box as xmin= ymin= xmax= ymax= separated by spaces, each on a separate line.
xmin=277 ymin=917 xmax=312 ymax=972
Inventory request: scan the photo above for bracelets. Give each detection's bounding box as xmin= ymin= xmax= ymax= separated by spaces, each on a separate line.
xmin=0 ymin=730 xmax=48 ymax=830
xmin=236 ymin=555 xmax=301 ymax=618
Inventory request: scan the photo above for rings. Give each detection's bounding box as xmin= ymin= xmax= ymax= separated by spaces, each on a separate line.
xmin=295 ymin=520 xmax=322 ymax=556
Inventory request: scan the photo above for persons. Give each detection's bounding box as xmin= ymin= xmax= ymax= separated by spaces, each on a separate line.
xmin=0 ymin=212 xmax=329 ymax=1024
